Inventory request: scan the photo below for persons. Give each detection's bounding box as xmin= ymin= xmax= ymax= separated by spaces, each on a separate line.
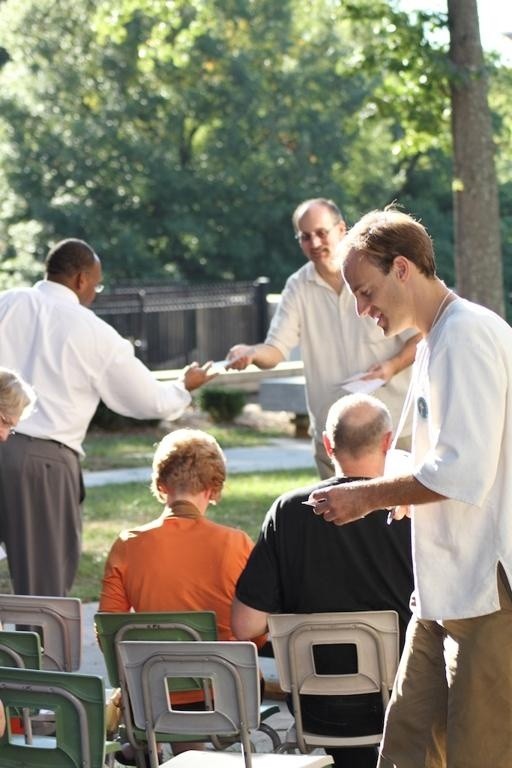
xmin=0 ymin=368 xmax=37 ymax=738
xmin=0 ymin=237 xmax=220 ymax=649
xmin=97 ymin=426 xmax=272 ymax=767
xmin=226 ymin=391 xmax=414 ymax=767
xmin=308 ymin=197 xmax=511 ymax=767
xmin=223 ymin=198 xmax=422 ymax=478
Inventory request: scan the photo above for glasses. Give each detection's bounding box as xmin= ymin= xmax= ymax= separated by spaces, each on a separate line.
xmin=294 ymin=221 xmax=341 ymax=242
xmin=0 ymin=413 xmax=17 ymax=438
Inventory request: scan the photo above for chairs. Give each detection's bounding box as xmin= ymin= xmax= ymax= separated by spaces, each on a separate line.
xmin=1 ymin=592 xmax=402 ymax=767
